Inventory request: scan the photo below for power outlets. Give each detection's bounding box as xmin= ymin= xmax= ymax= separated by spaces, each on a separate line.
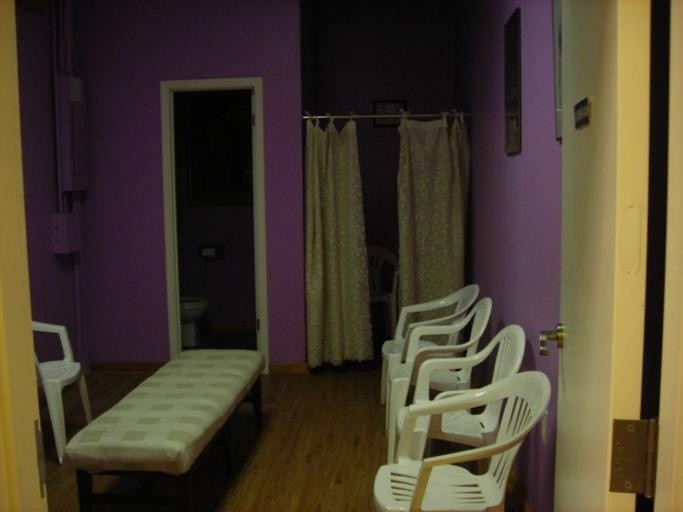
xmin=575 ymin=98 xmax=591 ymax=129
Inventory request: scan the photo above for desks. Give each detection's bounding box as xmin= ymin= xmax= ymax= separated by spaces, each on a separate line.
xmin=64 ymin=349 xmax=266 ymax=512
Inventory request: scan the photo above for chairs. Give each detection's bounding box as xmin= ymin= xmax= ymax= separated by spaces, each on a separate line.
xmin=371 ymin=371 xmax=552 ymax=512
xmin=387 ymin=297 xmax=494 ymax=465
xmin=395 ymin=323 xmax=527 ymax=460
xmin=32 ymin=320 xmax=93 ymax=464
xmin=380 ymin=283 xmax=480 ymax=406
